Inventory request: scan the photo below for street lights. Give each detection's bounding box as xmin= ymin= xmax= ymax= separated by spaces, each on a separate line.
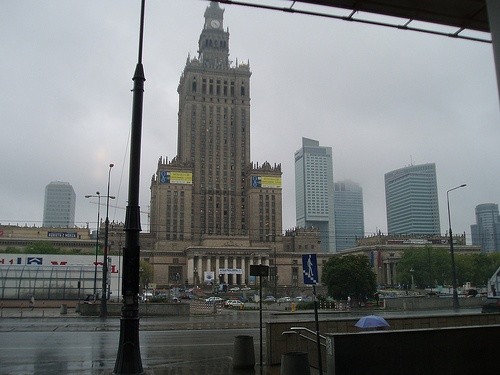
xmin=265 ymin=234 xmax=285 ymax=300
xmin=101 ymin=164 xmax=114 ymax=316
xmin=447 ymin=184 xmax=467 ymax=308
xmin=84 ymin=192 xmax=115 ymax=297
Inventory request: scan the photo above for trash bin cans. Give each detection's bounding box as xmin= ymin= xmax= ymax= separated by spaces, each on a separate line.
xmin=234 ymin=335 xmax=256 ymax=367
xmin=291 ymin=303 xmax=297 ymax=311
xmin=281 ymin=351 xmax=311 ymax=375
xmin=60 ymin=304 xmax=66 ymax=314
xmin=76 ymin=302 xmax=81 ymax=311
xmin=240 ymin=304 xmax=245 ymax=310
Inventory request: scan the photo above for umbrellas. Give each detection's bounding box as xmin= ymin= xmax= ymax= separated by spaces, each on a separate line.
xmin=355 ymin=316 xmax=391 ymax=329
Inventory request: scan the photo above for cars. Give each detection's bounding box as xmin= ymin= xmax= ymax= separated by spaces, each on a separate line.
xmin=264 ymin=296 xmax=319 ymax=303
xmin=149 ymin=294 xmax=177 ymax=303
xmin=481 ymin=297 xmax=500 ymax=314
xmin=205 ymin=297 xmax=223 ymax=304
xmin=224 ymin=300 xmax=244 ymax=309
